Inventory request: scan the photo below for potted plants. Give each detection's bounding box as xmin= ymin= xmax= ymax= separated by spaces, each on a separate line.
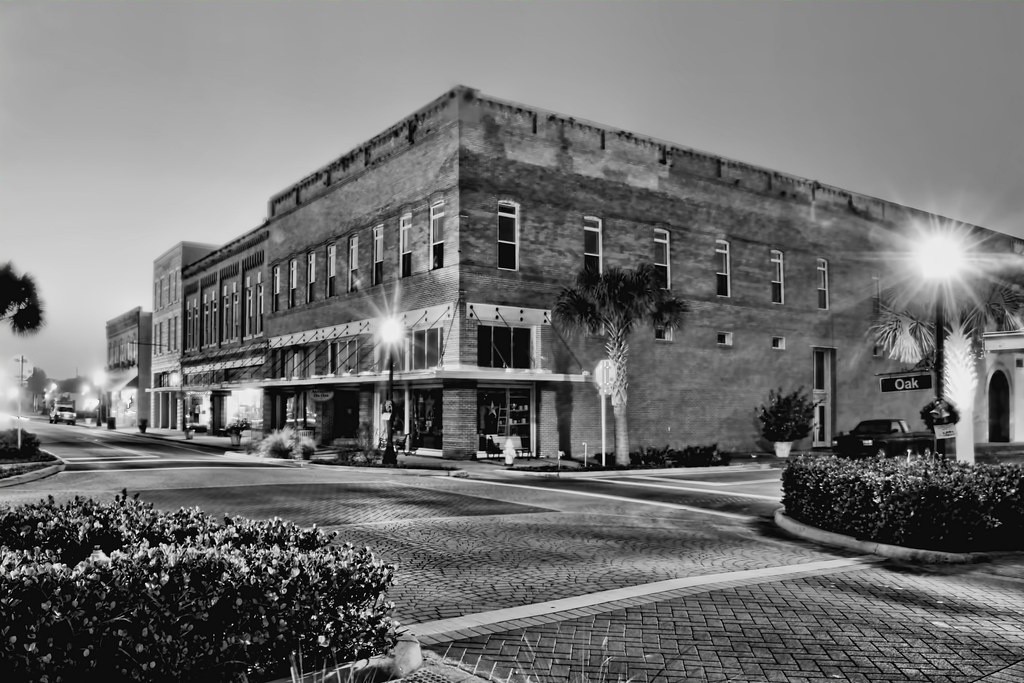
xmin=753 ymin=384 xmax=825 ymax=458
xmin=224 ymin=418 xmax=251 ymax=446
xmin=185 ymin=425 xmax=195 ymax=439
xmin=138 ymin=419 xmax=147 ymax=434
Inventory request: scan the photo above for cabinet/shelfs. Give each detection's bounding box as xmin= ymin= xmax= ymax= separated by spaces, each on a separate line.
xmin=504 ymin=395 xmax=530 ymax=438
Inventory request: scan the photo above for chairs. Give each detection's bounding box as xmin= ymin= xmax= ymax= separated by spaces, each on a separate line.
xmin=486 ymin=439 xmax=503 ymax=462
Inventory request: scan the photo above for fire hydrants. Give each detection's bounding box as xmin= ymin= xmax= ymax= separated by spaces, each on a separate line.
xmin=503 ymin=439 xmax=518 ymax=466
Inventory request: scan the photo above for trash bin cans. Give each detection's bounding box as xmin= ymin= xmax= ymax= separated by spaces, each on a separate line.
xmin=107 ymin=417 xmax=115 ymax=429
xmin=296 ymin=429 xmax=314 ymax=455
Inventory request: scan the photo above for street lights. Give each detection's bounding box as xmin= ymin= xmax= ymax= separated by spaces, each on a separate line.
xmin=927 ymin=240 xmax=953 ymax=396
xmin=380 ymin=318 xmax=405 ymax=466
xmin=95 ymin=369 xmax=105 ymax=425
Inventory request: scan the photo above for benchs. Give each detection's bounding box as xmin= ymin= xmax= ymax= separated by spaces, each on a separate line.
xmin=378 ymin=434 xmax=410 ymax=456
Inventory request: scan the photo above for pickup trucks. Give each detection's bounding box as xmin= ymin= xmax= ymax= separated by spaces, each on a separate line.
xmin=832 ymin=419 xmax=934 ymax=461
xmin=49 ymin=403 xmax=76 ymax=425
